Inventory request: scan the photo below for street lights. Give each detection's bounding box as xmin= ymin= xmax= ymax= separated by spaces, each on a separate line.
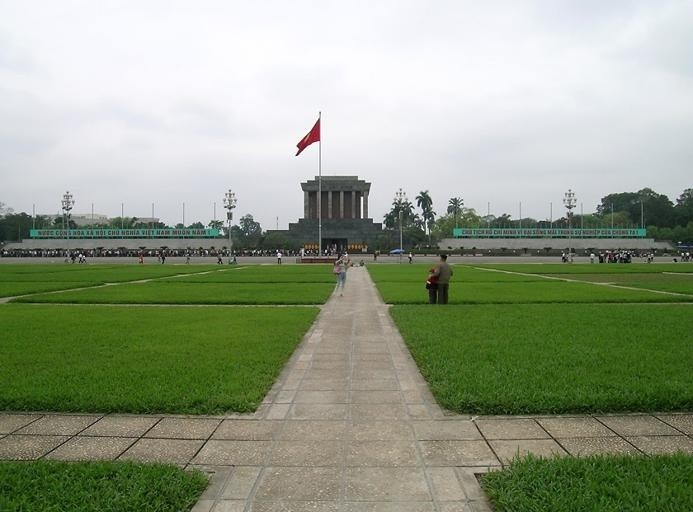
xmin=62 ymin=192 xmax=75 ymax=246
xmin=223 ymin=190 xmax=237 ymax=253
xmin=393 ymin=188 xmax=408 ymax=259
xmin=563 ymin=190 xmax=577 ymax=251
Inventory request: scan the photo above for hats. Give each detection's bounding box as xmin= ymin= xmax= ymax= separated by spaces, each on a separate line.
xmin=429 ymin=268 xmax=437 ymax=273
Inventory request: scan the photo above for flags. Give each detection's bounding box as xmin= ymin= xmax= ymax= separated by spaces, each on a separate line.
xmin=295 ymin=118 xmax=320 ymax=156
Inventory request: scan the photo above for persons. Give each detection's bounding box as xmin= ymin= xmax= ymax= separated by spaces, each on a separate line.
xmin=411 ymin=252 xmax=416 ymax=263
xmin=1 ymin=246 xmax=364 ymax=257
xmin=63 ymin=252 xmax=86 ymax=264
xmin=374 ymin=249 xmax=378 ymax=261
xmin=437 ymin=254 xmax=454 ymax=304
xmin=276 ymin=251 xmax=282 ymax=264
xmin=137 ymin=252 xmax=143 ymax=264
xmin=408 ymin=252 xmax=412 ymax=264
xmin=425 ymin=268 xmax=438 ymax=304
xmin=337 ymin=251 xmax=364 ymax=266
xmin=157 ymin=252 xmax=166 ymax=264
xmin=333 ymin=254 xmax=348 ymax=297
xmin=185 ymin=255 xmax=189 ymax=264
xmin=560 ymin=248 xmax=693 ymax=264
xmin=216 ymin=254 xmax=238 ymax=264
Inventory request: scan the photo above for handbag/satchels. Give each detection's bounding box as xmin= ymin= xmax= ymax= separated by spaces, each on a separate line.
xmin=333 ymin=266 xmax=341 ymax=274
